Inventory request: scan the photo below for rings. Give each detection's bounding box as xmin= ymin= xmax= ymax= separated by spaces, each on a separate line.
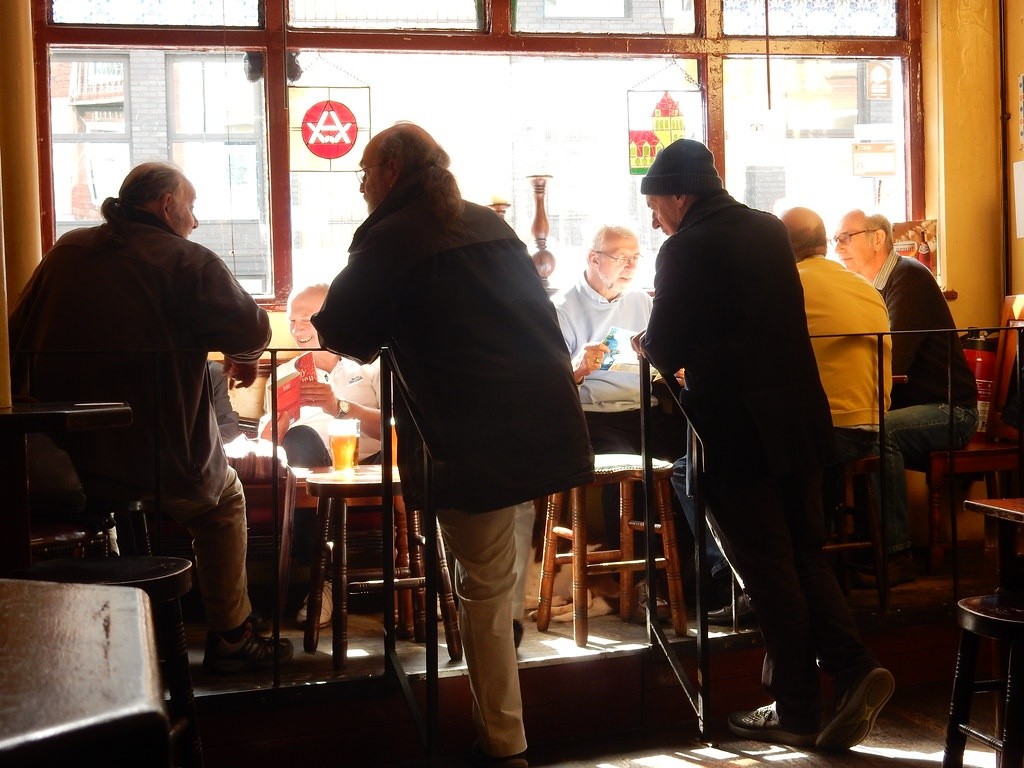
xmin=595 ymin=359 xmax=599 ymax=364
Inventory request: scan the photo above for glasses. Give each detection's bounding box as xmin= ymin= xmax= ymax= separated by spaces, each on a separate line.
xmin=594 ymin=250 xmax=647 ymax=266
xmin=830 ymin=229 xmax=878 ymax=247
xmin=355 ymin=164 xmax=382 ymax=183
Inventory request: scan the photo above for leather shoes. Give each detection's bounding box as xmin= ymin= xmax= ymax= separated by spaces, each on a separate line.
xmin=706 ymin=595 xmax=754 ymax=623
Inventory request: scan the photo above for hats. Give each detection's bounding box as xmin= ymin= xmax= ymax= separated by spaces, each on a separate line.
xmin=641 ymin=138 xmax=723 ymax=195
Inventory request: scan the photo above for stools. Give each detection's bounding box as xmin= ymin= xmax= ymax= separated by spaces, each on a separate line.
xmin=55 ymin=559 xmax=198 ymax=768
xmin=536 ymin=454 xmax=688 ymax=647
xmin=822 ymin=450 xmax=889 ymax=606
xmin=944 ymin=594 xmax=1024 ymax=768
xmin=302 ymin=470 xmax=463 ymax=671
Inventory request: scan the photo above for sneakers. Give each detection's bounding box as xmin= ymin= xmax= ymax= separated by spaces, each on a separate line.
xmin=814 ymin=661 xmax=894 ymax=749
xmin=202 ymin=623 xmax=293 ymax=671
xmin=634 ymin=580 xmax=670 ymax=622
xmin=729 ymin=701 xmax=815 ymax=746
xmin=297 ymin=582 xmax=334 ymax=628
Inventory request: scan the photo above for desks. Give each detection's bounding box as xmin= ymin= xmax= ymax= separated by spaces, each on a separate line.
xmin=0 ymin=399 xmax=133 ymax=582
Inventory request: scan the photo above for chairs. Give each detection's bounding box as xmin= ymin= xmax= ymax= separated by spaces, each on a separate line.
xmin=905 ymin=295 xmax=1024 ymax=573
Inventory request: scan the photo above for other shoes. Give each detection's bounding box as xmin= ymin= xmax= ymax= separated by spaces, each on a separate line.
xmin=468 ymin=740 xmax=528 ymax=767
xmin=854 ymin=553 xmax=915 ymax=587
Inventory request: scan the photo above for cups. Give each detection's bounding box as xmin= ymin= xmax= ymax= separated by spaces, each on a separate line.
xmin=893 ymin=242 xmax=917 ymax=257
xmin=329 ymin=419 xmax=360 ymax=472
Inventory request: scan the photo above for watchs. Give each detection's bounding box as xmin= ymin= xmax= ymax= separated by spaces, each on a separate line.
xmin=334 ymin=399 xmax=350 ymax=419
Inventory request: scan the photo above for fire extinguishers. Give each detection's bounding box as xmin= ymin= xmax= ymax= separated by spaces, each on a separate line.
xmin=959 ymin=327 xmax=1000 ymax=443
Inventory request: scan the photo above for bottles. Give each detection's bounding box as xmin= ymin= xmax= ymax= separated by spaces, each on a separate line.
xmin=918 ymin=231 xmax=931 ymax=270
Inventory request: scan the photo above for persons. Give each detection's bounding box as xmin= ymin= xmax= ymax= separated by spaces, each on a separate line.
xmin=549 ymin=203 xmax=976 ymax=625
xmin=631 ymin=139 xmax=895 ymax=750
xmin=206 ymin=360 xmax=238 ymax=445
xmin=257 ymin=281 xmax=443 ymax=628
xmin=311 ymin=121 xmax=596 ymax=768
xmin=8 ymin=162 xmax=293 ymax=676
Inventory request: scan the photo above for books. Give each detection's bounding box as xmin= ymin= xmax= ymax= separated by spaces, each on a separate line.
xmin=265 ymin=352 xmax=319 ymax=425
